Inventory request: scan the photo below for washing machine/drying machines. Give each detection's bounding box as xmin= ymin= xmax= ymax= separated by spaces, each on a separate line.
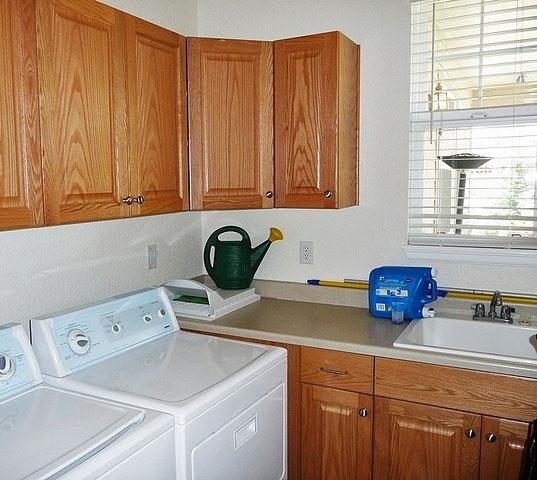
xmin=29 ymin=284 xmax=289 ymax=480
xmin=0 ymin=321 xmax=178 ymax=480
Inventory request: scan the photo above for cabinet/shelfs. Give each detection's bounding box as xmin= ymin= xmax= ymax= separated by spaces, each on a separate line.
xmin=184 ymin=35 xmax=275 ymax=212
xmin=35 ymin=0 xmax=190 ymax=226
xmin=1 ymin=0 xmax=46 ymax=232
xmin=374 ymin=358 xmax=537 ymax=480
xmin=272 ymin=31 xmax=360 ymax=207
xmin=299 ymin=345 xmax=374 ymax=480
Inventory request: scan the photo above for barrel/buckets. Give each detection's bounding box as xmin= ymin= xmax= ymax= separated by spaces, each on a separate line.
xmin=368 ymin=266 xmax=438 ymax=318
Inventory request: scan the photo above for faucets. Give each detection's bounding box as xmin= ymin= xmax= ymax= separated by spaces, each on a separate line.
xmin=488 ymin=290 xmax=502 ymax=321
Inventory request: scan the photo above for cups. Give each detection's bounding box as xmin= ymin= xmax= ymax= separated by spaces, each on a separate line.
xmin=390 ymin=302 xmax=407 ymax=324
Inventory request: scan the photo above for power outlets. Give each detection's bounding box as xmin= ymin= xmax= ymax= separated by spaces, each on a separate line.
xmin=299 ymin=241 xmax=314 ymax=264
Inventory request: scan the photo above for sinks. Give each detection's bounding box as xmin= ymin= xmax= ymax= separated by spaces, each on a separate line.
xmin=392 ymin=309 xmax=537 ymax=365
